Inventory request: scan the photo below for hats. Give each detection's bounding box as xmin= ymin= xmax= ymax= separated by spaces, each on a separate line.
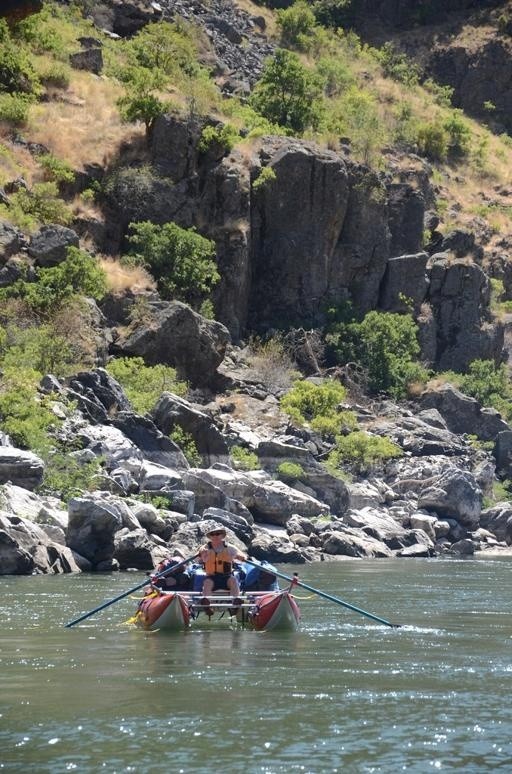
xmin=206 ymin=523 xmax=226 ymax=538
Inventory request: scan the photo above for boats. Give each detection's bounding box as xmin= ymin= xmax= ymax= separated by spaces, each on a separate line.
xmin=137 ymin=548 xmax=301 ymax=634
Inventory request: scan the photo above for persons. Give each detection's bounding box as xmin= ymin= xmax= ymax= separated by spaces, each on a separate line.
xmin=198 ymin=527 xmax=245 ymax=616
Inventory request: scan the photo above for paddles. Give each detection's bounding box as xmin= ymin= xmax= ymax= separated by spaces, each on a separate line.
xmin=244 ymin=557 xmax=402 ymax=628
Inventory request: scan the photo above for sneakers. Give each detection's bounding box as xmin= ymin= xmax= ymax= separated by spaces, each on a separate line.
xmin=200 ymin=598 xmax=214 ymax=615
xmin=230 ymin=598 xmax=242 ymax=615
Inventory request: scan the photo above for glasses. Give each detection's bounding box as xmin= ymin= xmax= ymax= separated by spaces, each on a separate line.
xmin=210 ymin=532 xmax=222 ymax=536
